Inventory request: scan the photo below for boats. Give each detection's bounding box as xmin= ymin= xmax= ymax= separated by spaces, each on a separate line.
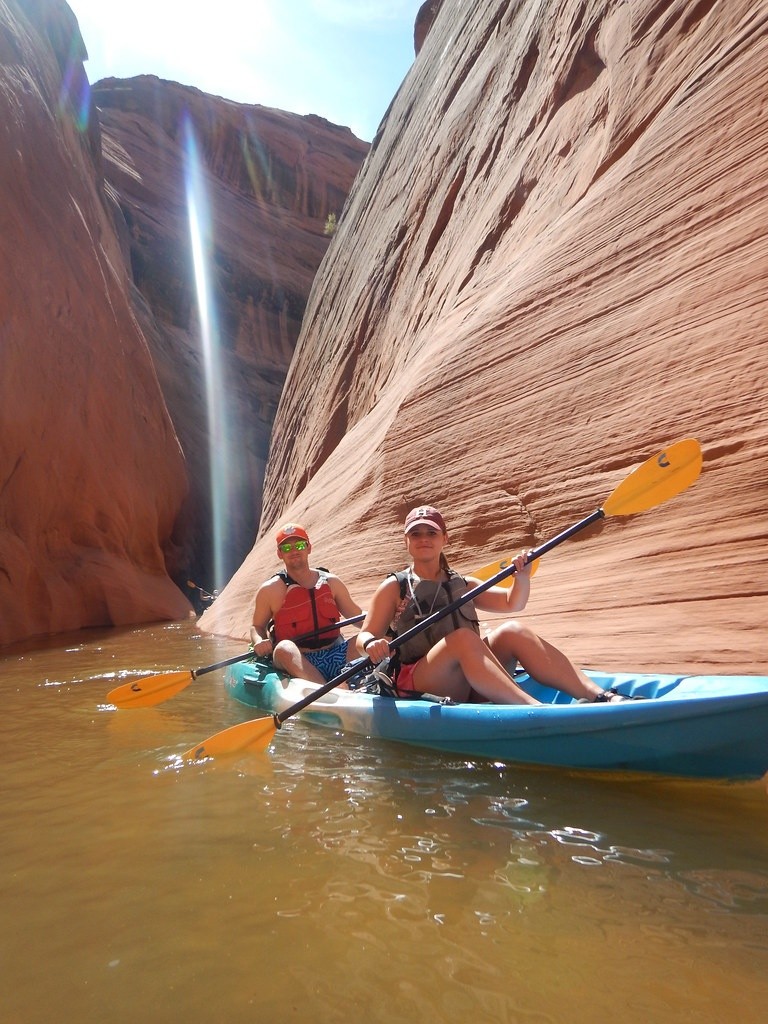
xmin=222 ymin=653 xmax=768 ymax=786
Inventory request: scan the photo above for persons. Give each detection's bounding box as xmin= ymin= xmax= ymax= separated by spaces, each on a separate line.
xmin=355 ymin=505 xmax=646 ymax=705
xmin=253 ymin=523 xmax=369 ymax=688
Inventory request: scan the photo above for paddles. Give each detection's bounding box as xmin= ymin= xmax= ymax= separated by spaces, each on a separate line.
xmin=186 ymin=437 xmax=703 ymax=761
xmin=107 ymin=559 xmax=540 ymax=706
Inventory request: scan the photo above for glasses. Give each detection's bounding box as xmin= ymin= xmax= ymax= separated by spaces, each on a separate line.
xmin=279 ymin=540 xmax=308 ymax=553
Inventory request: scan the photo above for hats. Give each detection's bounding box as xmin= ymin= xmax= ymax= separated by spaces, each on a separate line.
xmin=276 ymin=523 xmax=310 ymax=546
xmin=404 ymin=505 xmax=446 ymax=535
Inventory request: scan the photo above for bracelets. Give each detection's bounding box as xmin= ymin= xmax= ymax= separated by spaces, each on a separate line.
xmin=363 ymin=637 xmax=379 ymax=652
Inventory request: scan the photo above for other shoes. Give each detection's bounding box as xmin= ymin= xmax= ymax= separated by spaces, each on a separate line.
xmin=595 ymin=688 xmax=645 ymax=702
